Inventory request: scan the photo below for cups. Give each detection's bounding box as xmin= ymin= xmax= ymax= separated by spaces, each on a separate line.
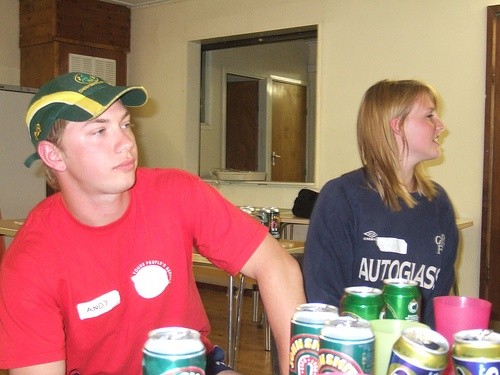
xmin=433 ymin=296 xmax=492 ymax=375
xmin=370 ymin=318 xmax=430 ymax=375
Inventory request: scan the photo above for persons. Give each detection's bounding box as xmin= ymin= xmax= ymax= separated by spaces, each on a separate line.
xmin=302 ymin=79 xmax=459 ymax=331
xmin=0 ymin=72 xmax=307 ymax=375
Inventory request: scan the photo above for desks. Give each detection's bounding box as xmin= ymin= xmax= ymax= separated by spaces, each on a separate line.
xmin=0 ymin=207 xmax=472 ymax=371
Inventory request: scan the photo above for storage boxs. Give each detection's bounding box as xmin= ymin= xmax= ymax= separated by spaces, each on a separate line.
xmin=19 ymin=0 xmax=131 ymax=91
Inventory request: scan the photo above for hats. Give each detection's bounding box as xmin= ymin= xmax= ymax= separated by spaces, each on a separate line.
xmin=25 ymin=72 xmax=149 ymax=168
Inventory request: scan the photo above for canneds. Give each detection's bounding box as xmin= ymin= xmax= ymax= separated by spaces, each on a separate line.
xmin=288 ymin=303 xmax=340 ymax=375
xmin=142 ymin=326 xmax=206 ymax=375
xmin=381 ymin=278 xmax=421 ymax=323
xmin=316 ymin=318 xmax=376 ymax=375
xmin=339 ymin=286 xmax=387 ymax=322
xmin=386 ymin=327 xmax=450 ymax=375
xmin=257 ymin=208 xmax=280 ymax=240
xmin=451 ymin=328 xmax=500 ymax=375
